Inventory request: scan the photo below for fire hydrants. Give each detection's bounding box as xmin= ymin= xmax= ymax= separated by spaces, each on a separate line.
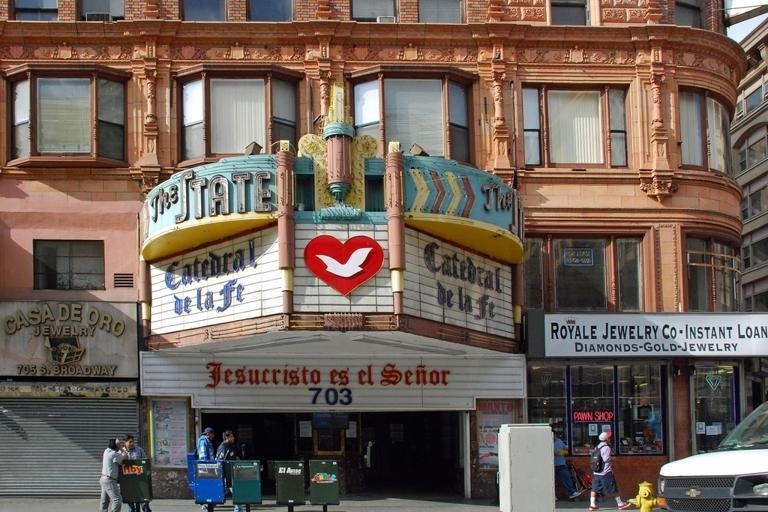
xmin=625 ymin=480 xmax=657 ymax=512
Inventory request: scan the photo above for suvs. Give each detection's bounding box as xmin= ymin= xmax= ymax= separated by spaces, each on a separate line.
xmin=657 ymin=401 xmax=768 ymax=512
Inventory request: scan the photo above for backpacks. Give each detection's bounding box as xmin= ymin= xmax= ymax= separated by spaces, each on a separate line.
xmin=591 ymin=444 xmax=609 ymax=472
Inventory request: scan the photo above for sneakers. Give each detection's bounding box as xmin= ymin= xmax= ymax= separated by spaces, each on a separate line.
xmin=618 ymin=501 xmax=631 ymax=509
xmin=589 ymin=504 xmax=598 ymax=510
xmin=569 ymin=491 xmax=582 ymax=498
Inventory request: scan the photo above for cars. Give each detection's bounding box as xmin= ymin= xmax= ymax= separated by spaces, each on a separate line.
xmin=550 ymin=421 xmax=596 ymax=443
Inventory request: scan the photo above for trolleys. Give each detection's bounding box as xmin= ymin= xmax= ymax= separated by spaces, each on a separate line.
xmin=565 ymin=458 xmax=593 ymax=503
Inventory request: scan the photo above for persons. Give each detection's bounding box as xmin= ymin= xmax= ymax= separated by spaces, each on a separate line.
xmin=117 ymin=436 xmax=151 ymax=512
xmin=99 ymin=439 xmax=128 ymax=512
xmin=202 ymin=431 xmax=243 ymax=512
xmin=194 ymin=427 xmax=216 ymax=506
xmin=552 ymin=430 xmax=581 ymax=501
xmin=589 ymin=432 xmax=631 ymax=511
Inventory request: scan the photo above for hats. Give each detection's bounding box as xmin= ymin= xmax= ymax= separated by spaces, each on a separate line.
xmin=201 ymin=427 xmax=213 ymax=434
xmin=599 ymin=431 xmax=612 ymax=440
